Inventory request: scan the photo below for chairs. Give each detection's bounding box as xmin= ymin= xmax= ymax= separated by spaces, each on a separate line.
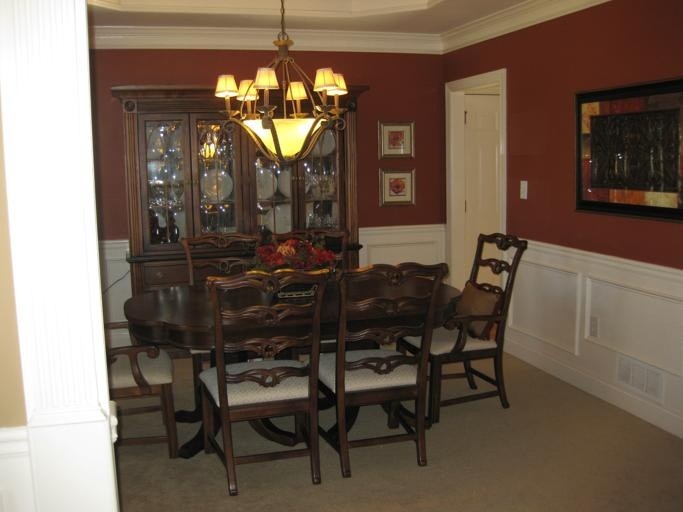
xmin=104 ymin=344 xmax=180 ymax=461
xmin=199 ymin=264 xmax=332 ymax=496
xmin=394 ymin=231 xmax=528 ymax=425
xmin=313 ymin=261 xmax=451 ymax=477
xmin=266 ymin=227 xmax=350 ymax=272
xmin=177 ymin=233 xmax=262 ymax=287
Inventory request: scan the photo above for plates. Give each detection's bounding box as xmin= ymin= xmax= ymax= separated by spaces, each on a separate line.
xmin=264 ymin=203 xmax=293 ymax=235
xmin=200 ymin=168 xmax=233 ymax=202
xmin=278 ymin=166 xmax=310 ymax=198
xmin=256 ymin=168 xmax=278 ymax=200
xmin=309 ymin=129 xmax=336 ymax=157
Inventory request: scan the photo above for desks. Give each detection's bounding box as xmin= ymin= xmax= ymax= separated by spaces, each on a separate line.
xmin=123 ymin=275 xmax=462 ymax=459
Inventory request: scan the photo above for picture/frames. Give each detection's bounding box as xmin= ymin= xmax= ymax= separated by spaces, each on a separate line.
xmin=378 ymin=120 xmax=415 ymax=160
xmin=378 ymin=168 xmax=416 ymax=208
xmin=576 ymin=78 xmax=683 ymax=224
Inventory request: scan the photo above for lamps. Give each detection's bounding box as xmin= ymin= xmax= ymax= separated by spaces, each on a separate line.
xmin=214 ymin=1 xmax=350 ymax=167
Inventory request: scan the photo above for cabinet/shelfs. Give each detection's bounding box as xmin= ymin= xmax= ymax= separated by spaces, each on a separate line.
xmin=109 ymin=83 xmax=370 ymax=298
